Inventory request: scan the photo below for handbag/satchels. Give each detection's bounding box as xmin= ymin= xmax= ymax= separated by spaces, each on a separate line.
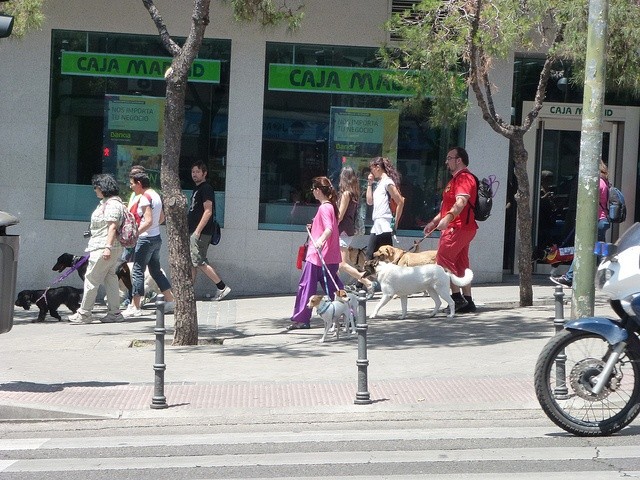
xmin=351 ymin=191 xmax=366 ymax=235
xmin=303 ymin=202 xmax=334 ymax=262
xmin=296 ymin=225 xmax=312 ymax=269
xmin=210 ymin=207 xmax=220 ymax=245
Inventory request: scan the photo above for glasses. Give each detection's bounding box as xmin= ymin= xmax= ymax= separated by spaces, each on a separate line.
xmin=446 ymin=156 xmax=459 ymax=160
xmin=94 ymin=184 xmax=98 ymax=189
xmin=311 ymin=188 xmax=316 ymax=192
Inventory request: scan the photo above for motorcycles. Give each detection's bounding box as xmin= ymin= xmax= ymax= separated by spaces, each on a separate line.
xmin=534 ymin=222 xmax=639 ymax=435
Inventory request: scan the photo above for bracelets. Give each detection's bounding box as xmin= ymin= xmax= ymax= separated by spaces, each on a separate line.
xmin=105 ymin=243 xmax=113 ymax=251
xmin=446 ymin=210 xmax=456 ymax=217
xmin=367 ymin=185 xmax=372 ymax=186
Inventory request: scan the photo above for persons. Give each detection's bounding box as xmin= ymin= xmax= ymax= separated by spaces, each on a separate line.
xmin=548 ymin=159 xmax=611 ymax=289
xmin=424 ymin=147 xmax=479 ymax=313
xmin=188 ymin=162 xmax=231 ymax=301
xmin=538 ymin=169 xmax=557 ymax=246
xmin=289 ymin=186 xmax=309 ymax=224
xmin=126 ymin=163 xmax=170 ymax=319
xmin=356 ymin=157 xmax=405 ymax=299
xmin=118 ymin=174 xmax=177 ymax=318
xmin=67 ymin=173 xmax=125 ymax=324
xmin=288 ymin=176 xmax=344 ymax=330
xmin=338 ymin=166 xmax=378 ymax=299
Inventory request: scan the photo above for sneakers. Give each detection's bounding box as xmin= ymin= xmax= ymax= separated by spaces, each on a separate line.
xmin=457 ymin=301 xmax=476 ymax=312
xmin=120 ymin=299 xmax=131 ymax=310
xmin=154 ymin=302 xmax=175 ymax=315
xmin=550 ymin=274 xmax=572 ymax=289
xmin=443 ymin=295 xmax=468 ymax=313
xmin=68 ymin=311 xmax=93 ymax=323
xmin=144 ymin=289 xmax=158 ymax=304
xmin=365 ymin=281 xmax=378 ymax=300
xmin=210 ymin=284 xmax=231 ymax=301
xmin=121 ymin=304 xmax=142 ymax=317
xmin=344 ymin=283 xmax=362 ymax=296
xmin=101 ymin=311 xmax=125 ymax=323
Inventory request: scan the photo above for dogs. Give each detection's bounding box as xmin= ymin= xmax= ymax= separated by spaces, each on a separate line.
xmin=347 ymin=245 xmax=368 ymax=271
xmin=374 ymin=244 xmax=439 ymax=297
xmin=53 ymin=253 xmax=131 ymax=303
xmin=115 ymin=262 xmax=170 ymax=308
xmin=333 ymin=289 xmax=360 ymax=335
xmin=306 ymin=294 xmax=350 ymax=342
xmin=358 ymin=260 xmax=474 ymax=319
xmin=15 ymin=285 xmax=84 ymax=322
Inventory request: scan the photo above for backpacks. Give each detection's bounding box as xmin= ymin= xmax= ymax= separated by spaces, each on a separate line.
xmin=454 ymin=171 xmax=492 ymax=224
xmin=103 ymin=199 xmax=139 ymax=248
xmin=599 ymin=177 xmax=626 ymax=223
xmin=129 ymin=190 xmax=165 ymax=228
xmin=384 ymin=185 xmax=406 ymax=225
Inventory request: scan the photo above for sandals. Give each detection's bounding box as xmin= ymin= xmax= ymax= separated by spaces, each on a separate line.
xmin=289 ymin=323 xmax=310 ymax=329
xmin=341 ymin=317 xmax=356 ymax=327
